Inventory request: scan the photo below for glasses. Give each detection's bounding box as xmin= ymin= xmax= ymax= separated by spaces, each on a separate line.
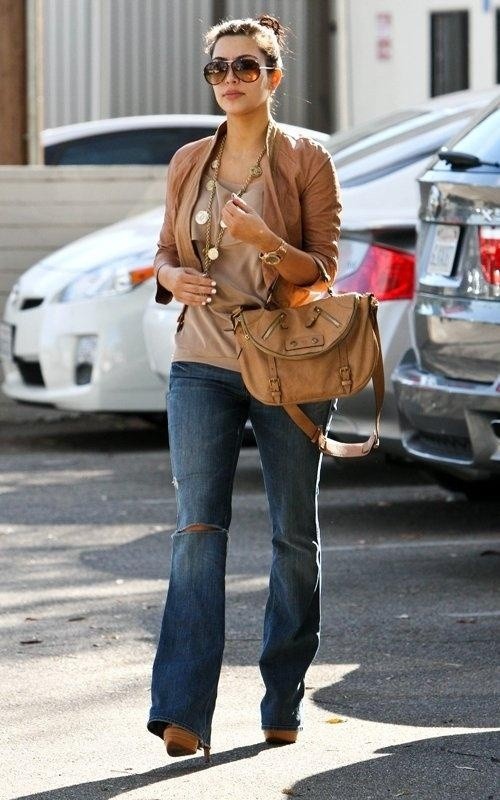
xmin=203 ymin=59 xmax=274 ymax=84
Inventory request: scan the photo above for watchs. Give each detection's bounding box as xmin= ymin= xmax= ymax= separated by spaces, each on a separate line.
xmin=257 ymin=238 xmax=288 ymax=267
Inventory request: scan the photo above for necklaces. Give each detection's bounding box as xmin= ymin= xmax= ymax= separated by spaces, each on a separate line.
xmin=200 ymin=133 xmax=267 ymax=298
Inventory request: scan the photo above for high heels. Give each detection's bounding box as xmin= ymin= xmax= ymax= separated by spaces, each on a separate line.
xmin=163 ymin=728 xmax=210 ymax=763
xmin=264 ymin=729 xmax=297 ymax=742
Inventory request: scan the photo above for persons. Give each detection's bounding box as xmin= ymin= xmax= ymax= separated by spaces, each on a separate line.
xmin=147 ymin=13 xmax=341 ymax=767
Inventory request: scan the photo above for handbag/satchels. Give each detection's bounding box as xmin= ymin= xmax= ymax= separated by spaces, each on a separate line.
xmin=233 ymin=293 xmax=379 ymax=406
